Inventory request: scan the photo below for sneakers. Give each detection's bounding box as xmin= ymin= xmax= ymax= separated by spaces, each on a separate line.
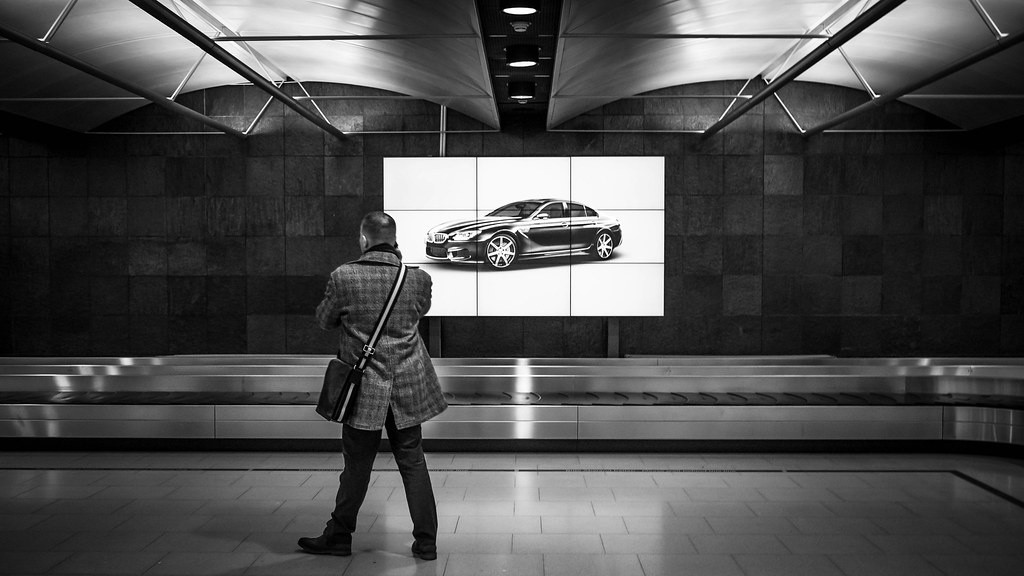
xmin=412 ymin=540 xmax=437 ymax=560
xmin=298 ymin=534 xmax=352 ymax=555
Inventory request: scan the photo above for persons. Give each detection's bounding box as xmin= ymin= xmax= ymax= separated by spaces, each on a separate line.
xmin=298 ymin=210 xmax=449 ymax=561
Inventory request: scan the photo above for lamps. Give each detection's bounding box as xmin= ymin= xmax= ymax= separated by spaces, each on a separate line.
xmin=508 ymin=81 xmax=536 ymax=100
xmin=499 ymin=0 xmax=542 ymax=16
xmin=506 ymin=43 xmax=539 ymax=68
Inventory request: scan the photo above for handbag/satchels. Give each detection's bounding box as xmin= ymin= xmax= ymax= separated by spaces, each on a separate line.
xmin=316 ymin=359 xmax=363 ymax=422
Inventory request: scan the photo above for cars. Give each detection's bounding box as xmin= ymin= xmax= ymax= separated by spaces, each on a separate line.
xmin=425 ymin=197 xmax=623 ymax=271
